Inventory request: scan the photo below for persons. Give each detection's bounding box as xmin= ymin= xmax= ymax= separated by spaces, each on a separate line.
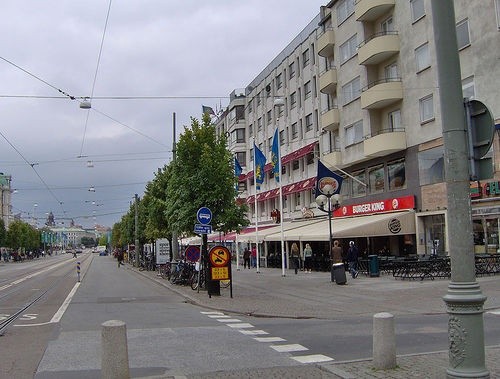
xmin=105 ymin=246 xmax=124 ymax=269
xmin=243 ymin=246 xmax=257 ymax=269
xmin=347 ymin=241 xmax=359 ymax=279
xmin=331 ymin=240 xmax=343 ymax=278
xmin=303 ymin=244 xmax=313 ymax=274
xmin=72 ymin=246 xmax=78 ymax=258
xmin=0 ymin=247 xmax=44 ymax=261
xmin=289 ymin=243 xmax=301 ymax=275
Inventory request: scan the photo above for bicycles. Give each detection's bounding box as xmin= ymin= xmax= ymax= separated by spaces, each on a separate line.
xmin=129 ymin=250 xmax=153 ymax=272
xmin=159 ymin=257 xmax=230 ymax=290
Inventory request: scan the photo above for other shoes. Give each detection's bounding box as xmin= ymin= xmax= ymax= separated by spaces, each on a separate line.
xmin=354 ymin=271 xmax=359 ymax=278
xmin=308 ymin=272 xmax=311 ymax=274
xmin=304 ymin=271 xmax=308 ymax=273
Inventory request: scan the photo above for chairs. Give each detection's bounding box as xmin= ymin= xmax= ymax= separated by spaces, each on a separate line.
xmin=358 ymin=252 xmax=500 ymax=281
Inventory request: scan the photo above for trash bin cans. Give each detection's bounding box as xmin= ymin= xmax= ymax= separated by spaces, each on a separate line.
xmin=260 ymin=257 xmax=266 ymax=267
xmin=368 ymin=254 xmax=380 ymax=277
xmin=206 ymin=269 xmax=221 ymax=297
xmin=333 ymin=262 xmax=347 ymax=284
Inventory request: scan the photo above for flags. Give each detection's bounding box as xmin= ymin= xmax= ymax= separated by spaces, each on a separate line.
xmin=271 ymin=127 xmax=279 ymax=183
xmin=314 ymin=159 xmax=342 ymax=197
xmin=253 ymin=144 xmax=267 ymax=193
xmin=234 ymin=158 xmax=243 ymax=193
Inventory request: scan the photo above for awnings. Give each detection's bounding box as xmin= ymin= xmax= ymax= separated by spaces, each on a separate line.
xmin=177 ymin=209 xmax=416 ymax=246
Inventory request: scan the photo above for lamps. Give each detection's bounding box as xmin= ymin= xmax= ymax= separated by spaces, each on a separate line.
xmin=87 ymin=160 xmax=95 ymax=167
xmin=87 ymin=184 xmax=95 ymax=192
xmin=78 ymin=96 xmax=91 ymax=110
xmin=273 ymin=96 xmax=285 ymax=107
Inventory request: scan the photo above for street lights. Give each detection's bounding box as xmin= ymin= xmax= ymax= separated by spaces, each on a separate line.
xmin=49 ymin=232 xmax=52 ymax=256
xmin=316 ymin=190 xmax=343 ymax=282
xmin=273 ymin=98 xmax=286 ymax=277
xmin=43 ymin=230 xmax=46 ymax=257
